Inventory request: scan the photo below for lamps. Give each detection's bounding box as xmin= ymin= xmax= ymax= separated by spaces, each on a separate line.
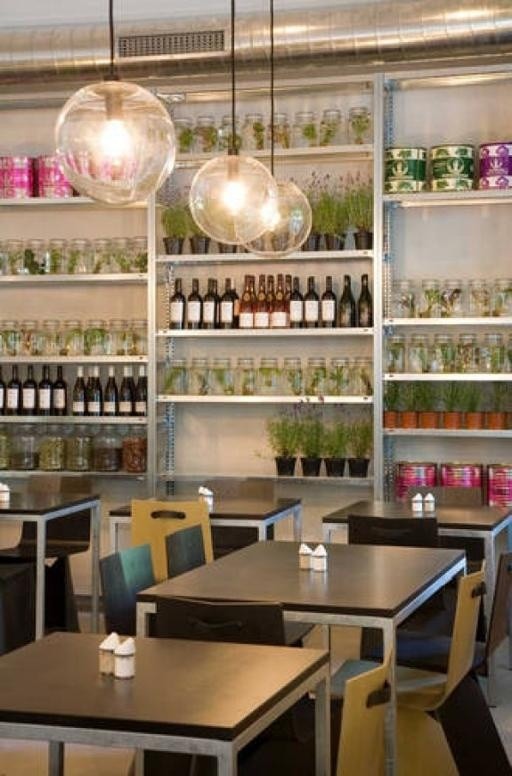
xmin=51 ymin=0 xmax=181 ymax=210
xmin=241 ymin=0 xmax=316 ymax=259
xmin=185 ymin=0 xmax=281 ymax=248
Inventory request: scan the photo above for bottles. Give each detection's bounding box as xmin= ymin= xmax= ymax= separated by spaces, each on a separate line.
xmin=0 ymin=315 xmax=148 ymax=474
xmin=96 ymin=629 xmax=136 ymax=681
xmin=384 ymin=276 xmax=512 ymax=371
xmin=1 ymin=236 xmax=147 ymax=274
xmin=172 ymin=104 xmax=372 ymax=157
xmin=296 ymin=538 xmax=327 ymax=572
xmin=0 ymin=482 xmax=11 ymax=503
xmin=412 ymin=491 xmax=436 ymax=514
xmin=199 ymin=486 xmax=215 ymax=510
xmin=169 ymin=272 xmax=374 ymax=397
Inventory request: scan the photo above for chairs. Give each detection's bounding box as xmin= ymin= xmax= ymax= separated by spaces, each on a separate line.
xmin=316 ymin=577 xmax=483 ymax=776
xmin=358 ymin=551 xmax=510 ymax=733
xmin=340 ymin=513 xmax=487 ymax=637
xmin=1 ymin=473 xmax=94 ymax=647
xmin=92 ymin=499 xmax=208 ymax=640
xmin=335 ymin=650 xmax=393 ymax=776
